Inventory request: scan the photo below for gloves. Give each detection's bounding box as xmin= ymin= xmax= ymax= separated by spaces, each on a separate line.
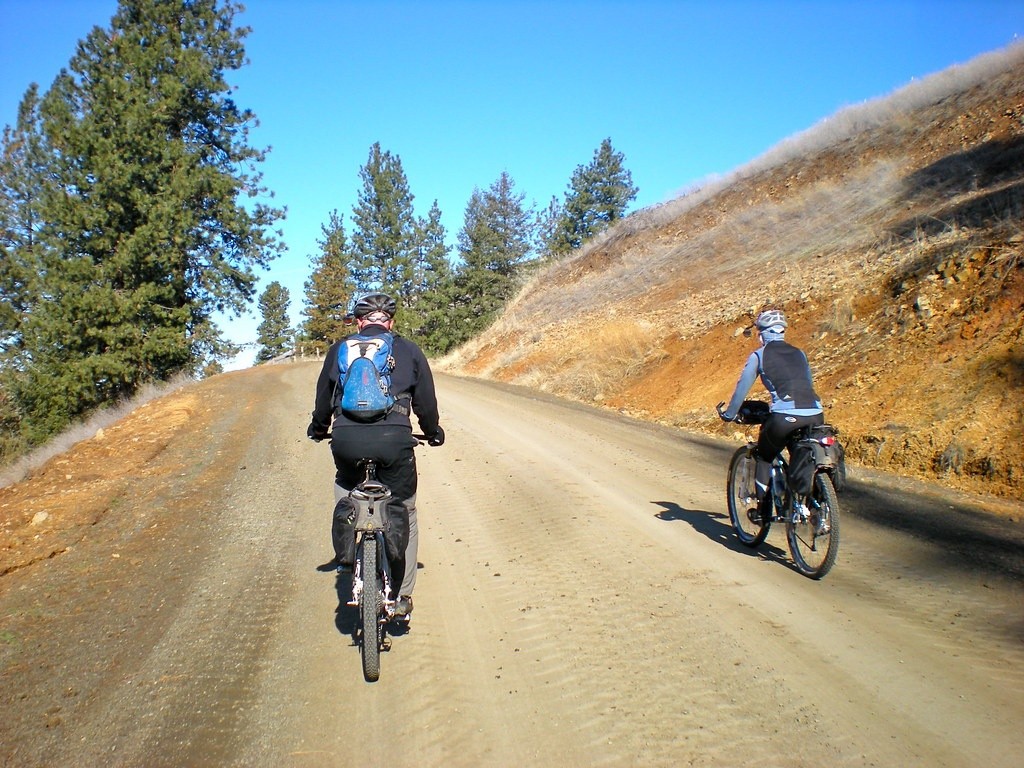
xmin=307 ymin=423 xmax=327 ymax=443
xmin=721 ymin=411 xmax=732 ymax=422
xmin=426 ymin=425 xmax=444 ymax=446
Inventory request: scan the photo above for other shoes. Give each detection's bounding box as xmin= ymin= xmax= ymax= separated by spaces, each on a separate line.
xmin=393 ymin=596 xmax=413 ymax=616
xmin=747 ymin=508 xmax=770 ymax=529
xmin=337 ymin=566 xmax=351 ymax=574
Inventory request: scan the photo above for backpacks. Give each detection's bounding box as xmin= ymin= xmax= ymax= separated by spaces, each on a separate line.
xmin=338 ymin=332 xmax=400 ymax=420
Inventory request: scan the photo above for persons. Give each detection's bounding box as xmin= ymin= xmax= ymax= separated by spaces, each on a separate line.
xmin=720 ymin=310 xmax=824 ymax=528
xmin=307 ymin=293 xmax=445 ymax=622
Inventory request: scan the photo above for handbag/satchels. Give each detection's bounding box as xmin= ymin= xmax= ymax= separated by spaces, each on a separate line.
xmin=382 ymin=496 xmax=409 ymax=562
xmin=333 ymin=497 xmax=356 ymax=566
xmin=826 ymin=443 xmax=846 ymax=493
xmin=786 ymin=443 xmax=817 ymax=495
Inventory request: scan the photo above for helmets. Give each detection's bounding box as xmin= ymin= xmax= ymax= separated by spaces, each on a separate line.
xmin=756 ymin=309 xmax=788 ymax=329
xmin=354 ymin=295 xmax=396 ymax=319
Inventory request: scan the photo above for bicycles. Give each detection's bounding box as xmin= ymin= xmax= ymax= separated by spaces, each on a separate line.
xmin=308 ymin=432 xmax=439 ymax=683
xmin=715 ymin=399 xmax=843 ymax=581
